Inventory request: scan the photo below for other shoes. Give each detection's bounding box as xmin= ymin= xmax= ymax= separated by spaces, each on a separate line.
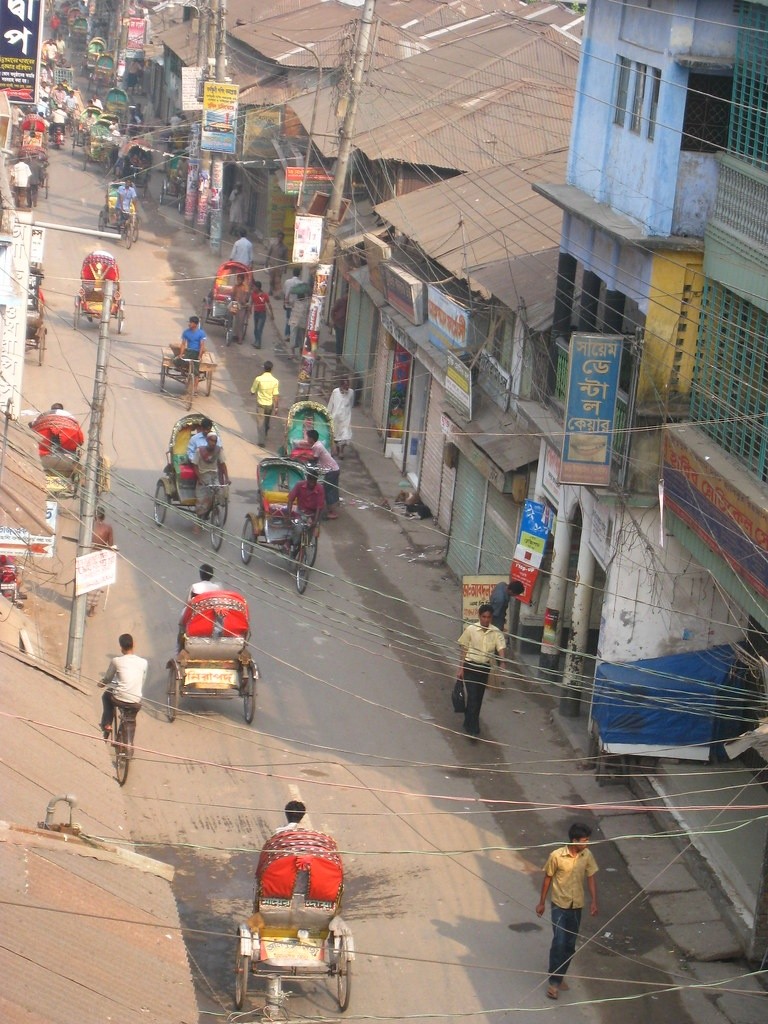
xmin=558 ymin=982 xmax=569 ymax=991
xmin=334 ymin=446 xmax=345 ymax=459
xmin=258 ymin=443 xmax=265 ymax=447
xmin=466 ymin=726 xmax=479 ymax=742
xmin=546 ymin=984 xmax=558 ymax=999
xmin=325 ymin=512 xmax=339 ymax=518
xmin=253 ymin=343 xmax=261 ymax=349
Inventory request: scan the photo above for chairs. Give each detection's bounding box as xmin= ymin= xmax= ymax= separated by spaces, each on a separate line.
xmin=83 ymin=277 xmax=115 ymax=298
xmin=216 ymin=283 xmax=247 ymax=303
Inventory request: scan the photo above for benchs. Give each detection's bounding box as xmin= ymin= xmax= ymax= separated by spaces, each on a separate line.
xmin=252 ymin=895 xmax=338 ymax=938
xmin=181 ymin=636 xmax=247 ymax=659
xmin=180 ymin=456 xmax=223 ymax=480
xmin=266 ymin=490 xmax=309 ymax=515
xmin=291 ymin=442 xmax=326 ymax=461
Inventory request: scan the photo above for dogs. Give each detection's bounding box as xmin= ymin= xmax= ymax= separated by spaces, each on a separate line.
xmin=395 ymin=490 xmax=430 ymax=521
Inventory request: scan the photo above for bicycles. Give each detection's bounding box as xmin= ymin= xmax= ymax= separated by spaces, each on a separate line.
xmin=102 ymin=680 xmax=135 ymax=785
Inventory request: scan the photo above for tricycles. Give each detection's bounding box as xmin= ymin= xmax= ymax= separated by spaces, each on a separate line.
xmin=241 ymin=457 xmax=322 ymax=592
xmin=153 ymin=414 xmax=232 ymax=551
xmin=201 ymin=261 xmax=256 ymax=348
xmin=232 ymin=831 xmax=354 ymax=1014
xmin=29 ymin=408 xmax=83 ymax=500
xmin=25 ymin=269 xmax=49 ymax=367
xmin=160 ymin=343 xmax=218 ymax=412
xmin=158 ymin=149 xmax=189 ymax=212
xmin=165 ymin=590 xmax=261 ymax=726
xmin=18 ymin=1 xmax=154 ymax=188
xmin=280 ymin=400 xmax=335 ymax=478
xmin=99 ymin=182 xmax=142 ymax=249
xmin=74 ymin=250 xmax=126 ymax=335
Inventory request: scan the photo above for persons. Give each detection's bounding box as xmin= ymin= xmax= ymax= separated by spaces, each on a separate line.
xmin=264 ymin=230 xmax=288 ymax=299
xmin=286 ymin=470 xmax=326 ymax=566
xmin=536 ymin=821 xmax=600 ymax=997
xmin=186 ymin=417 xmax=232 ymax=522
xmin=100 ymin=634 xmax=147 ymax=758
xmin=50 ymin=403 xmax=65 ymax=413
xmin=185 ymin=563 xmax=223 ymax=605
xmin=271 ymin=800 xmax=305 ymax=847
xmin=229 ymin=229 xmax=254 ymax=272
xmin=457 ymin=604 xmax=506 ymax=736
xmin=14 ymin=14 xmax=188 ymax=210
xmin=327 ymin=374 xmax=355 ymax=460
xmin=117 ymin=178 xmax=137 ymax=237
xmin=303 ymin=430 xmax=341 ymax=519
xmin=224 ymin=275 xmax=250 ymax=346
xmin=250 ymin=358 xmax=280 ymax=447
xmin=82 ymin=502 xmax=115 ymax=621
xmin=247 ymin=280 xmax=275 ymax=349
xmin=489 ymin=580 xmax=525 ymax=668
xmin=329 ymin=288 xmax=348 ymax=358
xmin=179 ymin=316 xmax=206 ymax=397
xmin=284 ymin=268 xmax=314 ymax=362
xmin=227 ymin=178 xmax=249 ymax=238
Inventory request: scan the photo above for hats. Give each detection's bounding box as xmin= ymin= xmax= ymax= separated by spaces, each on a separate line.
xmin=207 ymin=431 xmax=217 ymax=438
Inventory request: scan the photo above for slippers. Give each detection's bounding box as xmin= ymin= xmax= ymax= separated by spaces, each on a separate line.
xmin=126 ymin=749 xmax=135 ymax=757
xmin=101 ymin=723 xmax=112 ymax=732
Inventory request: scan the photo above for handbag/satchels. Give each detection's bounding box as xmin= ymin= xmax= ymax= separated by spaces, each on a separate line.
xmin=451 ymin=678 xmax=468 ymax=713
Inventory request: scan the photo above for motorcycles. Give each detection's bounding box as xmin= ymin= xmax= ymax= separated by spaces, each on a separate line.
xmin=0 ymin=554 xmax=29 ymax=609
